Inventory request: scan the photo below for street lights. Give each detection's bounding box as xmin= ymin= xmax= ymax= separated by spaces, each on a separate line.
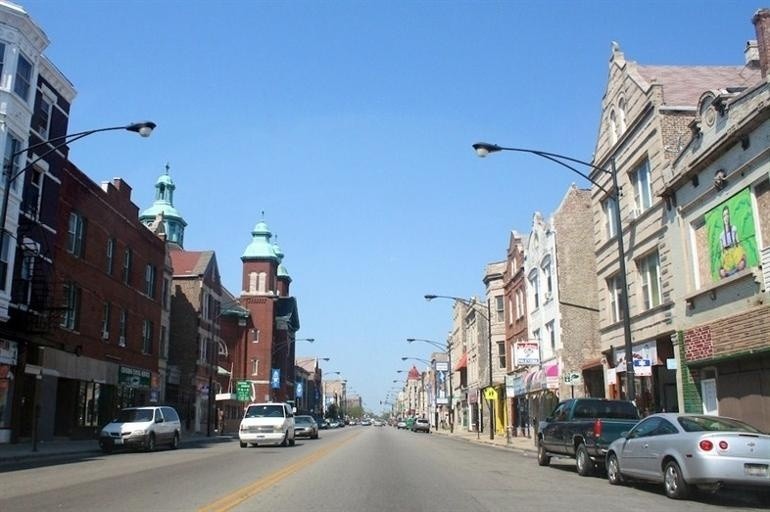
xmin=402 ymin=356 xmax=439 ymax=431
xmin=471 ymin=142 xmax=639 ymax=407
xmin=0 ymin=121 xmax=154 ymax=260
xmin=379 ymin=369 xmax=430 ymax=421
xmin=270 ymin=337 xmax=341 ymax=417
xmin=205 ymin=294 xmax=280 ymax=436
xmin=424 ymin=294 xmax=494 ymax=439
xmin=408 ymin=337 xmax=454 ymax=433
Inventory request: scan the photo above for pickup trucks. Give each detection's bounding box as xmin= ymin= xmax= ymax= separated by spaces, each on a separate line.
xmin=535 ymin=396 xmax=644 ymax=476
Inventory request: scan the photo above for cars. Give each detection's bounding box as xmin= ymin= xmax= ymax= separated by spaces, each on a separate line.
xmin=294 ymin=414 xmax=318 ymax=439
xmin=316 ymin=416 xmax=430 ymax=433
xmin=604 ymin=411 xmax=769 ymax=501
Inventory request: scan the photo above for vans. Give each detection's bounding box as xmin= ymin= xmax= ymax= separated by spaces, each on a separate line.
xmin=98 ymin=406 xmax=182 ymax=451
xmin=238 ymin=402 xmax=297 ymax=448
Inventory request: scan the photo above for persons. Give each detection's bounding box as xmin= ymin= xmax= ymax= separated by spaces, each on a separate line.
xmin=715 ymin=205 xmax=746 ymax=279
